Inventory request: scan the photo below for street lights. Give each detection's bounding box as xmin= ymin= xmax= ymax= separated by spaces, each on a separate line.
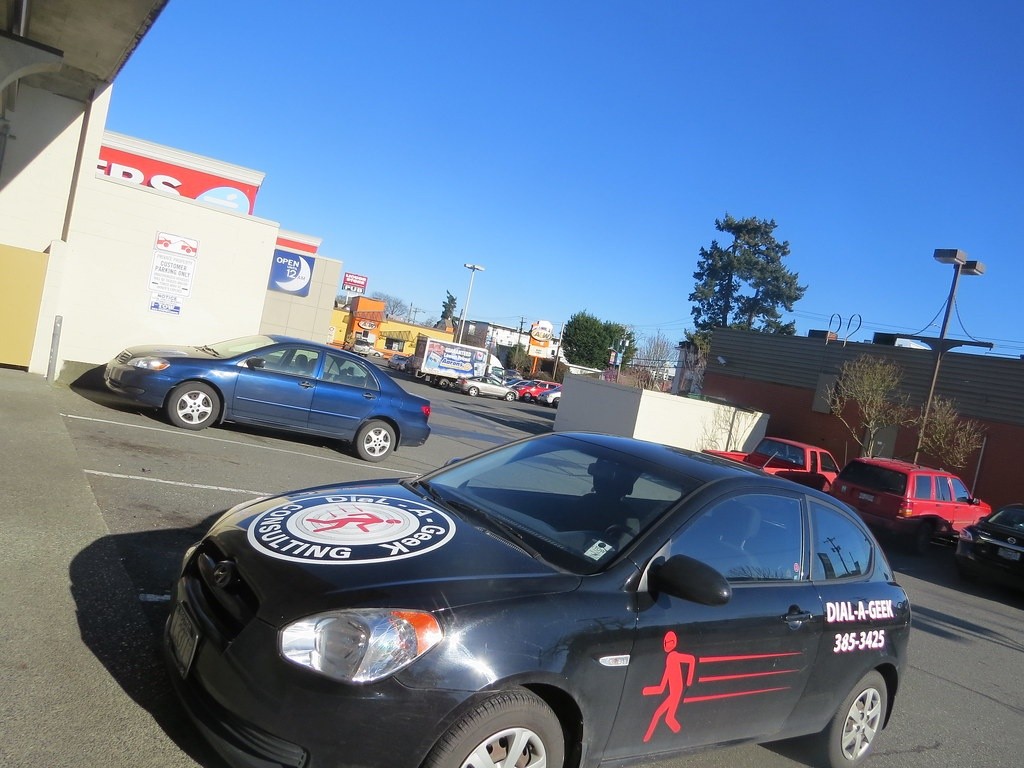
xmin=911 ymin=247 xmax=987 ymax=465
xmin=608 ymin=347 xmax=624 ymax=384
xmin=459 ymin=262 xmax=487 ymax=344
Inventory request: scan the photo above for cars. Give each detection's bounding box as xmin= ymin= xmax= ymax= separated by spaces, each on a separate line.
xmin=504 ymin=369 xmax=562 ymax=408
xmin=369 ymin=346 xmax=384 ymax=358
xmin=158 ymin=431 xmax=913 ymax=768
xmin=103 ymin=334 xmax=433 ymax=464
xmin=387 ymin=353 xmax=414 ymax=374
xmin=954 ymin=502 xmax=1024 ymax=591
xmin=454 ymin=375 xmax=520 ymax=402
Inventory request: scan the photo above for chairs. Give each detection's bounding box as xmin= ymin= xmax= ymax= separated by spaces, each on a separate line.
xmin=291 ymin=354 xmax=308 ymax=372
xmin=692 ymin=498 xmax=762 ymax=574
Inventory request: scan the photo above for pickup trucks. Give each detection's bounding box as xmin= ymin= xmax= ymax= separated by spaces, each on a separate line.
xmin=701 ymin=435 xmax=841 ymax=496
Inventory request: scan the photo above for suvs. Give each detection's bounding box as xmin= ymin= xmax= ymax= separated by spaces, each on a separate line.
xmin=350 ymin=338 xmax=370 ymax=357
xmin=828 ymin=455 xmax=993 ymax=559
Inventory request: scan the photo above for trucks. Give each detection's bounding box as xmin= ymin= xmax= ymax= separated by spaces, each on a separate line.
xmin=410 ymin=334 xmax=504 ymax=390
